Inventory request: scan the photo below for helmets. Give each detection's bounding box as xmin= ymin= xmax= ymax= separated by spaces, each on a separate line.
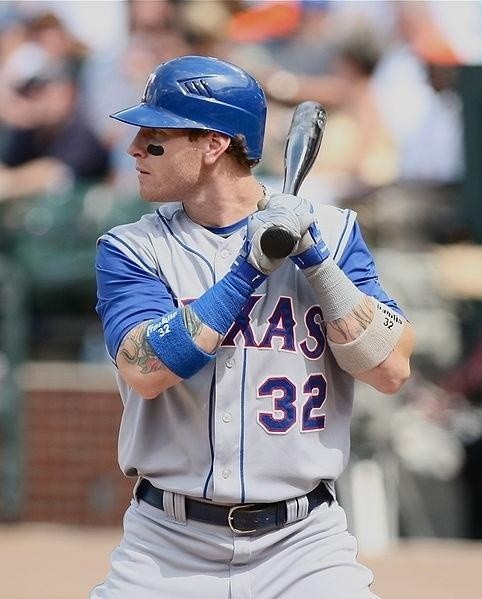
xmin=109 ymin=55 xmax=266 ymax=159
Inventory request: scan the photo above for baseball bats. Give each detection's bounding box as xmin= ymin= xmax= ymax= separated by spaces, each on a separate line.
xmin=260 ymin=102 xmax=326 ymax=261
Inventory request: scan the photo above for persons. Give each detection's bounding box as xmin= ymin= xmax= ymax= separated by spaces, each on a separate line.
xmin=0 ymin=1 xmax=482 ymax=520
xmin=89 ymin=56 xmax=415 ymax=599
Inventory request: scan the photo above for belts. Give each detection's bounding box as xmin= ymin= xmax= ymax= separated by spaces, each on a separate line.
xmin=137 ymin=480 xmax=332 ymax=534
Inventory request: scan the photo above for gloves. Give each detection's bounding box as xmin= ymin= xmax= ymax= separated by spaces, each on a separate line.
xmin=230 ymin=194 xmax=329 ymax=287
